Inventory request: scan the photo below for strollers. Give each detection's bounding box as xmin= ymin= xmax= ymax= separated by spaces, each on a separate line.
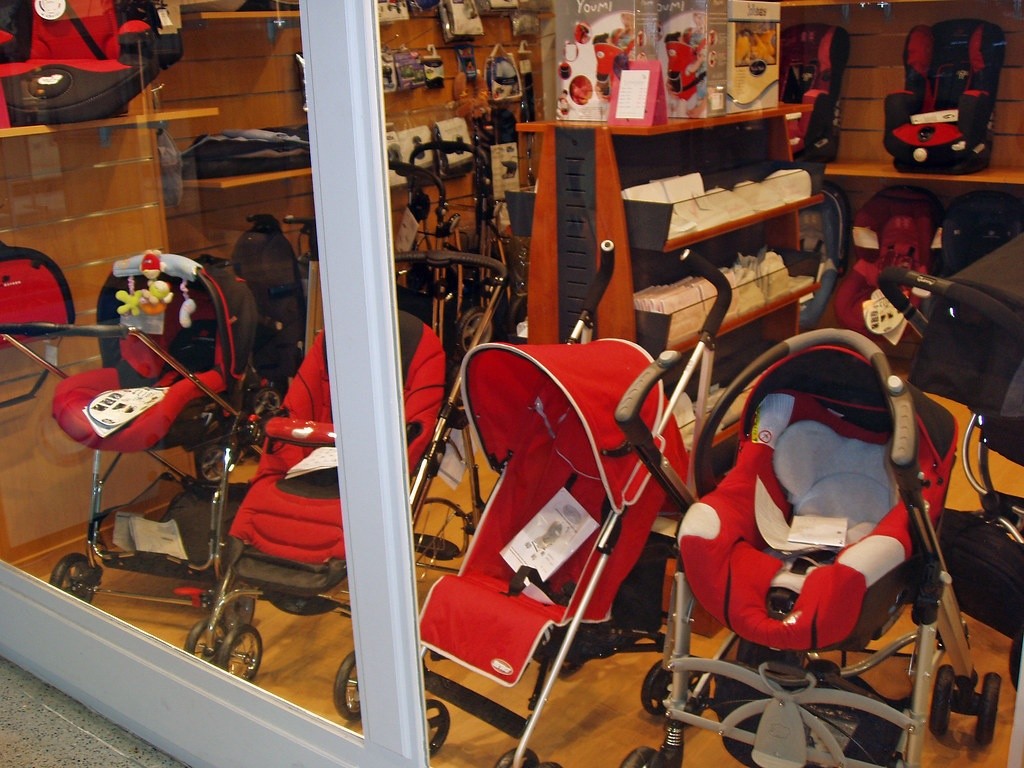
xmin=875 ymin=230 xmax=1021 ymax=692
xmin=188 ymin=135 xmax=508 ymax=700
xmin=610 ymin=328 xmax=1004 ymax=768
xmin=1 ymin=214 xmax=320 ymax=608
xmin=330 ymin=239 xmax=733 ymax=768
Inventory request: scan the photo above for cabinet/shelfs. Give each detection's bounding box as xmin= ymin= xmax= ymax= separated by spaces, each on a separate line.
xmin=504 ymin=103 xmax=824 ymax=401
xmin=0 ymin=10 xmax=311 ymax=189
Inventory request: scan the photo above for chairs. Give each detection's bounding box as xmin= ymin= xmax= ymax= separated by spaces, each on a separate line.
xmin=0 ymin=1 xmax=184 ymax=130
xmin=883 ymin=18 xmax=1007 ymax=176
xmin=777 ymin=23 xmax=850 ymax=166
xmin=792 ymin=186 xmax=1024 ymax=330
